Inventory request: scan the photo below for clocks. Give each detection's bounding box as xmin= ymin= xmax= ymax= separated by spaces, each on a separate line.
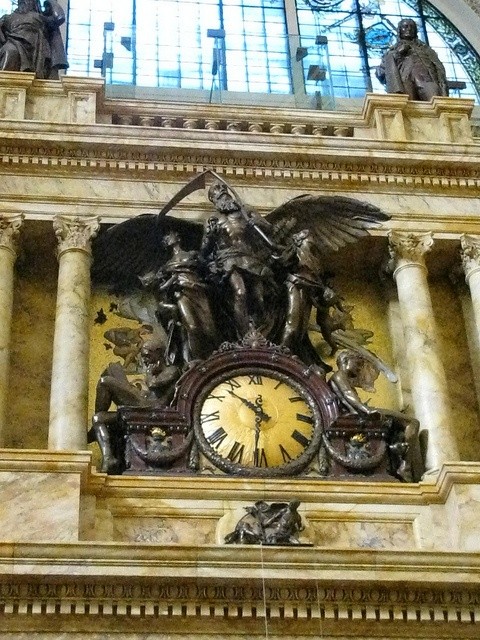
xmin=190 ymin=350 xmax=323 ymax=476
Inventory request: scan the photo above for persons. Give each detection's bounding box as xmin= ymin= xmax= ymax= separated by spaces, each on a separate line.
xmin=262 ymin=500 xmax=306 ymax=543
xmin=88 ymin=339 xmax=181 ymax=475
xmin=328 ymin=349 xmax=420 ymax=483
xmin=374 ymin=19 xmax=449 ymax=102
xmin=199 ymin=179 xmax=282 ymax=344
xmin=138 ymin=231 xmax=218 ymax=361
xmin=0 ymin=0 xmax=69 ymax=80
